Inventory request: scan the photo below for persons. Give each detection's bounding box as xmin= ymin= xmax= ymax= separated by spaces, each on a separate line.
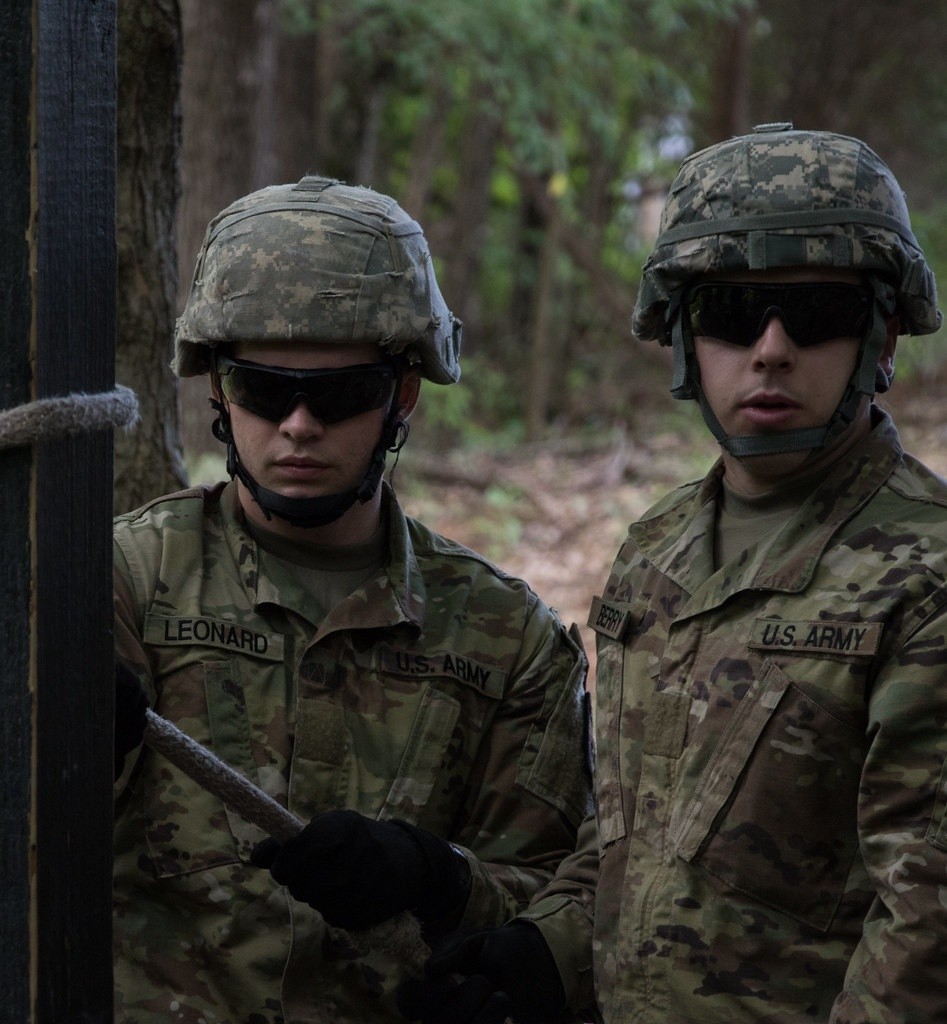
xmin=426 ymin=122 xmax=947 ymax=1024
xmin=113 ymin=174 xmax=594 ymax=1021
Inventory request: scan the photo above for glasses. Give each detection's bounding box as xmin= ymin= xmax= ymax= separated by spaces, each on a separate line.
xmin=209 ymin=352 xmax=412 ymax=424
xmin=686 ymin=280 xmax=874 ymax=349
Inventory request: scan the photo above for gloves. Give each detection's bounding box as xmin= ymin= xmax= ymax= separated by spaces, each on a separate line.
xmin=112 ymin=659 xmax=148 ymax=787
xmin=249 ymin=808 xmax=467 ymax=930
xmin=394 ymin=919 xmax=552 ymax=1024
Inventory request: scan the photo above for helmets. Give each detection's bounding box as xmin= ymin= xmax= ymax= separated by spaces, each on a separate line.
xmin=170 ymin=175 xmax=462 ymax=386
xmin=631 ymin=122 xmax=943 ymax=348
